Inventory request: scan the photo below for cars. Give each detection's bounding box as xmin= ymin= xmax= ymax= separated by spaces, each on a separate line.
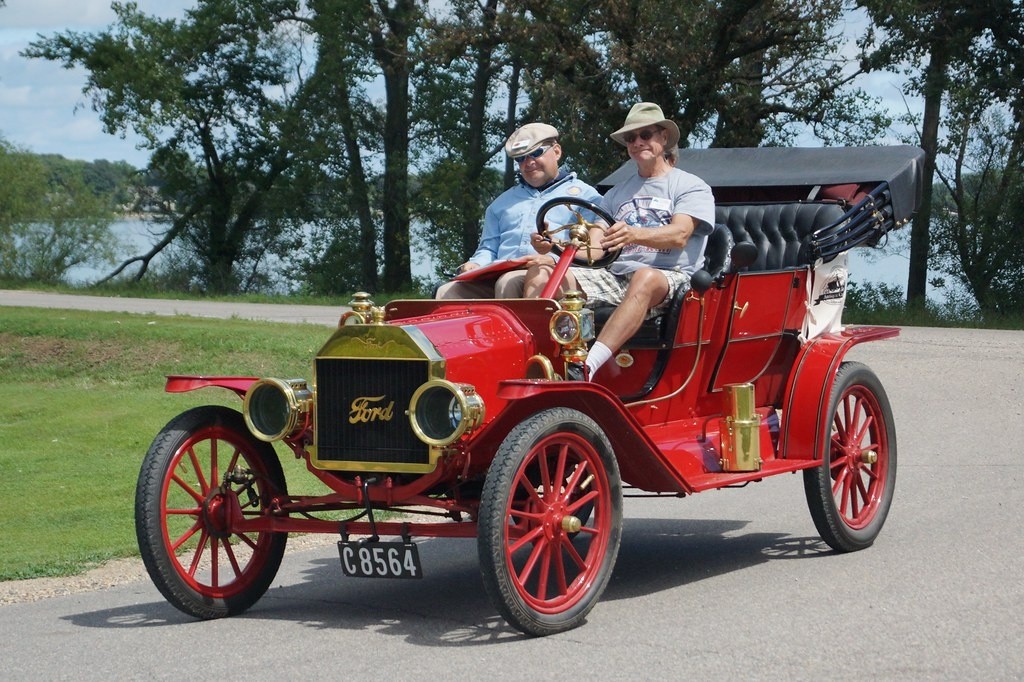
xmin=133 ymin=143 xmax=929 ymax=635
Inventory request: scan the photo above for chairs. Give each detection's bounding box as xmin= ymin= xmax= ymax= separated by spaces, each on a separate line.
xmin=590 ymin=222 xmax=734 ymax=353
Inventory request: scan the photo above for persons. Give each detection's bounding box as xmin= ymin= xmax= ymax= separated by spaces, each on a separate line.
xmin=435 ymin=123 xmax=606 ymax=300
xmin=522 ymin=101 xmax=717 ymax=382
